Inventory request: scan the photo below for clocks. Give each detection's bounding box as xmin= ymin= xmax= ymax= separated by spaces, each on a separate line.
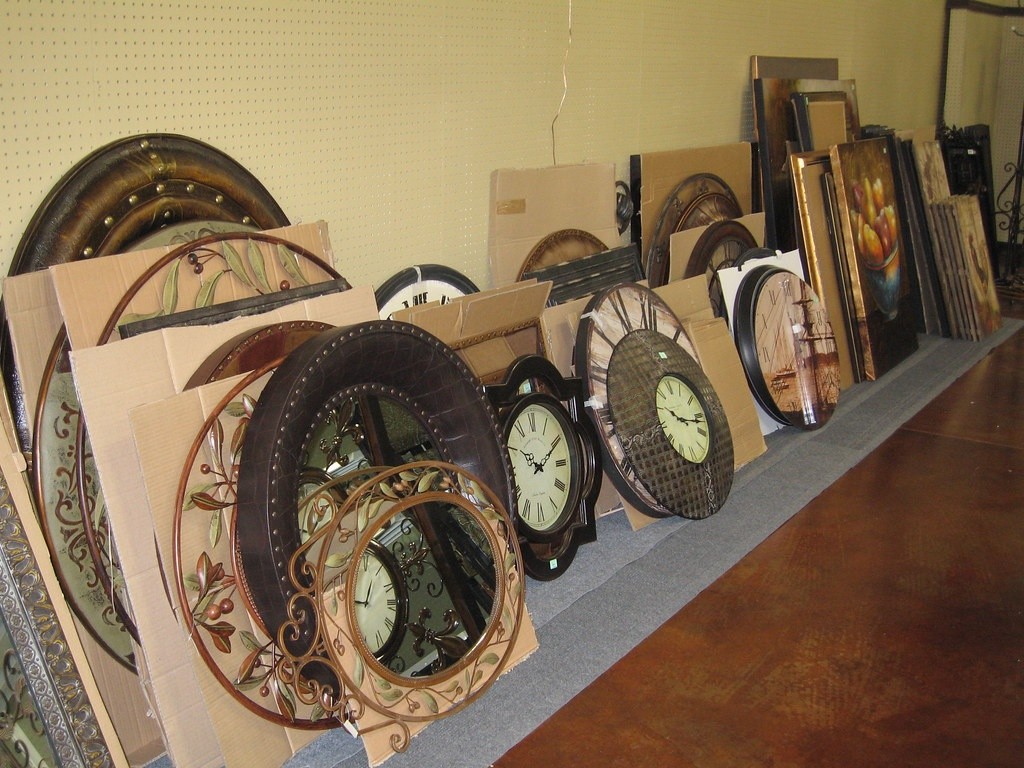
xmin=607 ymin=329 xmax=735 ymax=519
xmin=685 ymin=220 xmax=758 ymax=326
xmin=353 ymin=540 xmax=410 ymax=665
xmin=298 ymin=468 xmax=350 ymax=545
xmin=733 ymin=264 xmax=839 ymax=432
xmin=374 ymin=264 xmax=481 ymax=321
xmin=483 ymin=354 xmax=603 ymax=582
xmin=575 ymin=281 xmax=703 ymax=519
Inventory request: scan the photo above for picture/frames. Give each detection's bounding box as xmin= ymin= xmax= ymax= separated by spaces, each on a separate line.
xmin=789 ymin=149 xmax=855 ymax=394
